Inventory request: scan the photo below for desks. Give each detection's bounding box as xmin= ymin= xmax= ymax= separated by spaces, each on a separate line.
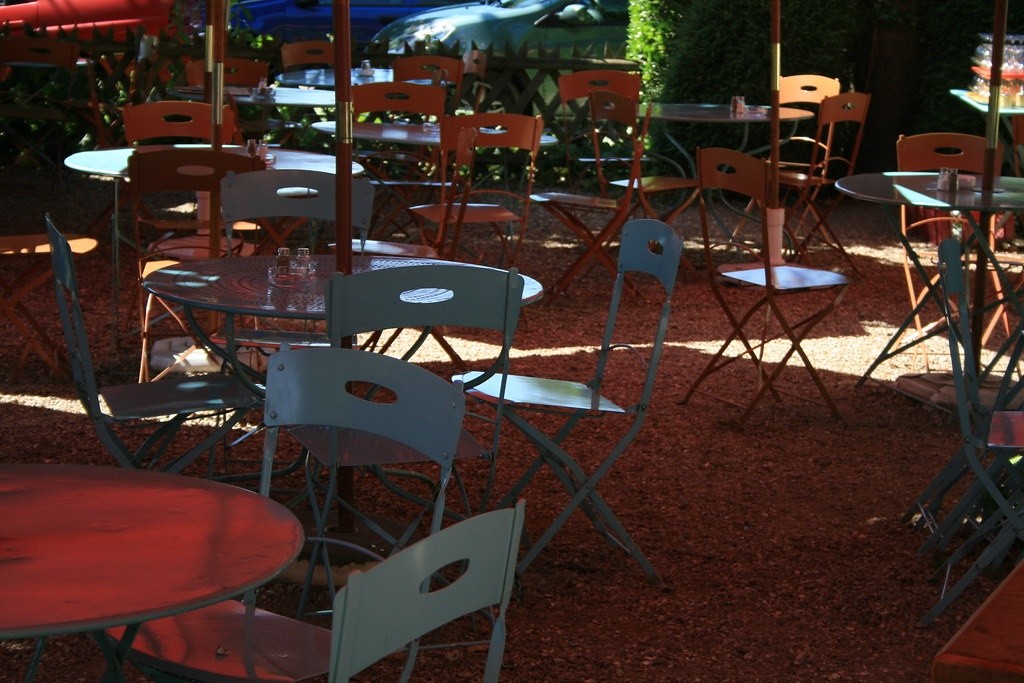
xmin=174 ymin=89 xmax=335 ymax=150
xmin=64 ymin=143 xmax=365 ymax=355
xmin=835 ymin=171 xmax=1023 ymax=414
xmin=949 ymin=89 xmax=1024 ymax=179
xmin=0 ymin=461 xmax=306 ymax=682
xmin=144 ymin=254 xmax=544 ymax=589
xmin=276 ymin=67 xmax=434 ymax=87
xmin=623 ymin=101 xmax=815 ymax=271
xmin=311 ymin=120 xmax=558 ymax=268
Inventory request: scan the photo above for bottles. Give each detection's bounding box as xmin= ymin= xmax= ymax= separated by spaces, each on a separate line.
xmin=248 ymin=78 xmax=280 ymax=103
xmin=730 ymin=96 xmax=745 ymax=112
xmin=432 ymin=68 xmax=449 ymax=88
xmin=361 ymin=59 xmax=370 ymax=69
xmin=947 ymin=168 xmax=958 ymax=191
xmin=296 ymin=247 xmax=309 ymax=273
xmin=258 ymin=139 xmax=267 ymax=156
xmin=276 ymin=248 xmax=290 ymax=271
xmin=246 ymin=139 xmax=256 ymax=157
xmin=969 ymin=33 xmax=1024 ymax=108
xmin=937 ymin=167 xmax=948 ymax=191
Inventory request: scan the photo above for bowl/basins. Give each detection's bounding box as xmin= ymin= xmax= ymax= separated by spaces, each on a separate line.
xmin=269 ymin=266 xmax=316 ymax=288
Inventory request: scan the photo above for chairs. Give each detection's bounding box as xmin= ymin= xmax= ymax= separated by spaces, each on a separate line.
xmin=0 ymin=40 xmax=1024 ymax=683
